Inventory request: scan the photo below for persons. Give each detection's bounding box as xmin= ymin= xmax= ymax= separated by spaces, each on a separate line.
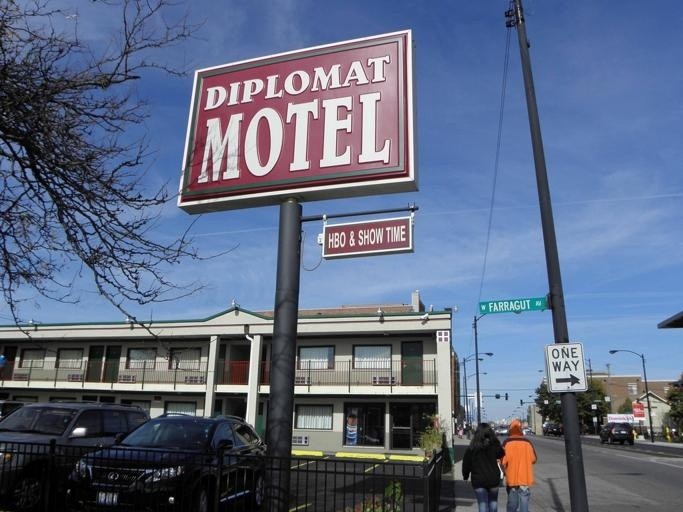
xmin=462 ymin=422 xmax=505 ymax=512
xmin=497 ymin=419 xmax=537 ymax=512
xmin=455 ymin=424 xmax=464 ymax=438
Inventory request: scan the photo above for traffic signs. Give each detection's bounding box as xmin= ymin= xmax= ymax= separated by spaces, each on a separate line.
xmin=546 ymin=342 xmax=589 ymax=393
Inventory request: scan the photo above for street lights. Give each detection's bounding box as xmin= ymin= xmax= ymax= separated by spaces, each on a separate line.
xmin=463 ymin=310 xmax=521 ymax=440
xmin=609 ymin=350 xmax=654 ymax=443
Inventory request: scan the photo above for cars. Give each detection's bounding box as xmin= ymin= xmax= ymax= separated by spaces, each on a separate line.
xmin=599 ymin=422 xmax=635 ymax=446
xmin=494 ymin=428 xmax=532 ymax=436
xmin=0 ymin=400 xmax=268 ymax=512
xmin=542 ymin=421 xmax=561 ymax=437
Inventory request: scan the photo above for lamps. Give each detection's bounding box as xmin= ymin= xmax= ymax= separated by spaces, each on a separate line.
xmin=232 ymin=299 xmax=240 ymax=310
xmin=124 ymin=315 xmax=133 ymax=329
xmin=29 ymin=319 xmax=37 ymax=331
xmin=452 ymin=305 xmax=458 ymax=313
xmin=419 ymin=313 xmax=429 ymax=321
xmin=376 ymin=307 xmax=384 ymax=316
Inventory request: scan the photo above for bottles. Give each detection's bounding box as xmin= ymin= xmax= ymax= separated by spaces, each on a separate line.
xmin=346 ymin=413 xmax=357 ymax=445
